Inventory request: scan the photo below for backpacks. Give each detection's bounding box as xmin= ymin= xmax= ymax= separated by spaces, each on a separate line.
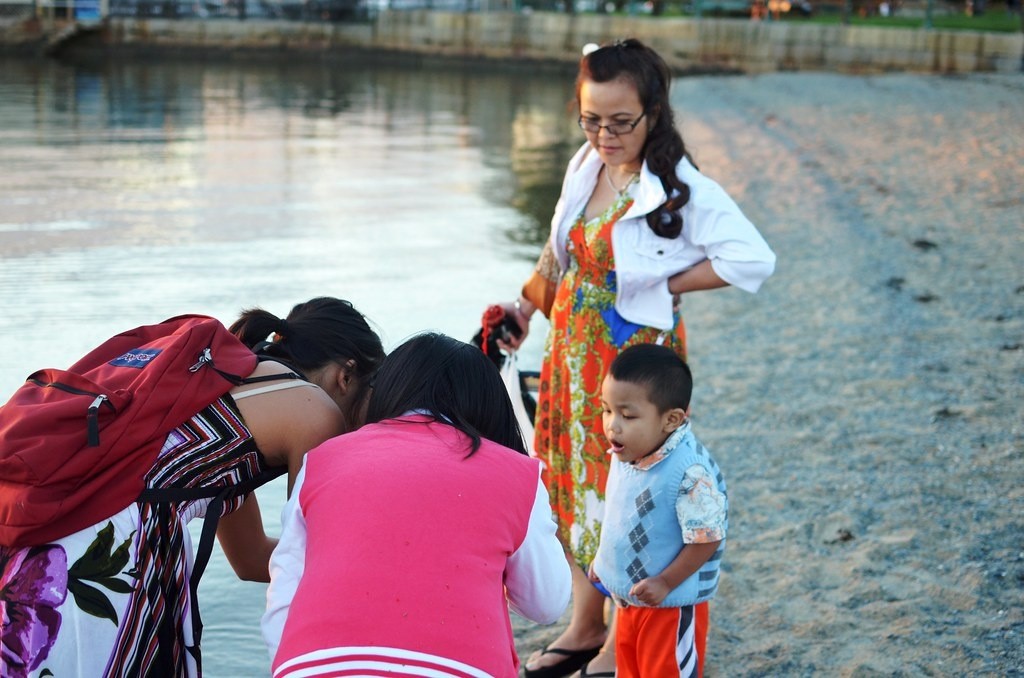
xmin=0 ymin=314 xmax=310 ymax=544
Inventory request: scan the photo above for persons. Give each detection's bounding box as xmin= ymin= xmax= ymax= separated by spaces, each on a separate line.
xmin=484 ymin=39 xmax=776 ymax=678
xmin=0 ymin=297 xmax=386 ymax=678
xmin=588 ymin=343 xmax=729 ymax=677
xmin=261 ymin=333 xmax=572 ymax=678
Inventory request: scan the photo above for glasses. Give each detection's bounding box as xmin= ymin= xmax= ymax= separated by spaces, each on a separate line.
xmin=578 ymin=106 xmax=646 ymax=135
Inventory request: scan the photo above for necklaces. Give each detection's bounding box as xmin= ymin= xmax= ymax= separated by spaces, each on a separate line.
xmin=606 ymin=164 xmax=636 ymax=201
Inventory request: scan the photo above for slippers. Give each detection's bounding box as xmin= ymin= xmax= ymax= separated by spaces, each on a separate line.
xmin=524 ymin=627 xmax=609 ymax=678
xmin=580 ymin=662 xmax=615 ymax=678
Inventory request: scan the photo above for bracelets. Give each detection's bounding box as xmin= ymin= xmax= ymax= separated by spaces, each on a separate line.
xmin=514 ymin=299 xmax=530 ymax=321
